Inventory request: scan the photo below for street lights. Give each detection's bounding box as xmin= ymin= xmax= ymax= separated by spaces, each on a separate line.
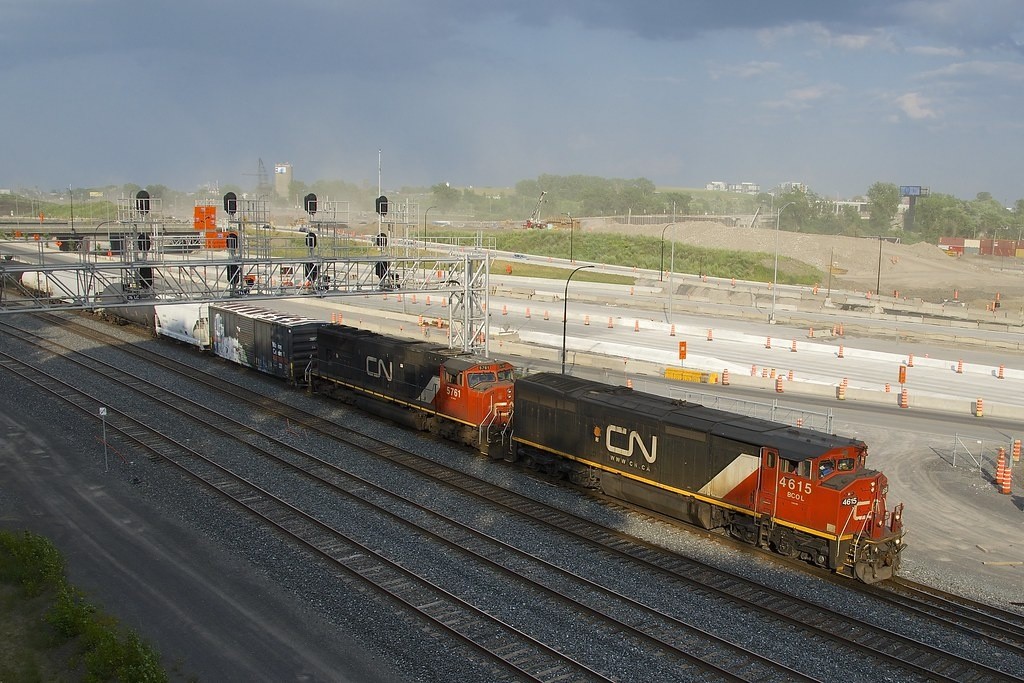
xmin=94 ymin=220 xmax=118 ymax=297
xmin=772 ymin=202 xmax=797 ymax=320
xmin=661 ymin=224 xmax=675 ymax=281
xmin=562 ymin=266 xmax=595 ymax=373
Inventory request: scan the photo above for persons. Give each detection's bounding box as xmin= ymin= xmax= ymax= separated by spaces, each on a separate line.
xmin=819 ymin=464 xmax=832 ymax=476
xmin=788 ymin=463 xmax=798 ymax=474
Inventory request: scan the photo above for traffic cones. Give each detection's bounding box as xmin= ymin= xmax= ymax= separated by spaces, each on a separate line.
xmin=994 ymin=437 xmax=1020 ymax=495
xmin=352 ymin=271 xmax=1024 ymax=415
xmin=330 ymin=310 xmax=343 ymax=325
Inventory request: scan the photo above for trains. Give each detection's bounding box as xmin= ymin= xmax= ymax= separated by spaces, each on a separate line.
xmin=1 ymin=243 xmax=902 ymax=585
